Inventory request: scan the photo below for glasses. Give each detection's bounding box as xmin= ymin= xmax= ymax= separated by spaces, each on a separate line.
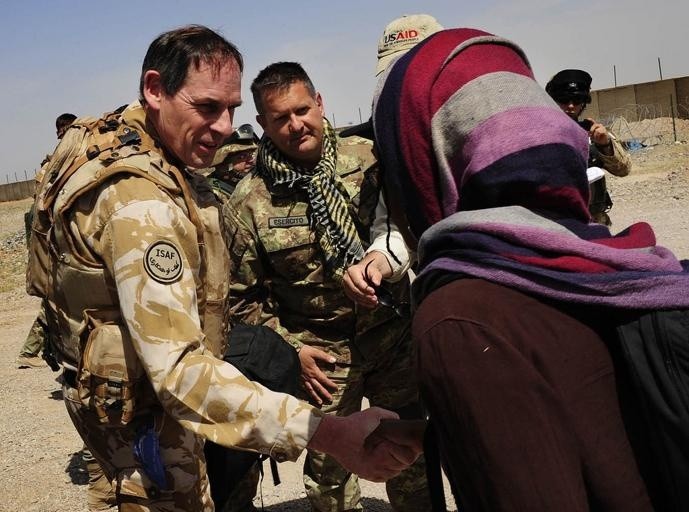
xmin=228 ymin=124 xmax=253 ymax=143
xmin=365 ymin=259 xmax=410 ymax=319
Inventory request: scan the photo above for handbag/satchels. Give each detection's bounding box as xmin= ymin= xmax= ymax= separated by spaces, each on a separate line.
xmin=225 ymin=323 xmax=303 ymax=401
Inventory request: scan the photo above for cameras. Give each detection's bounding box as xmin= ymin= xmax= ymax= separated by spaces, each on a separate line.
xmin=576 ymin=119 xmax=592 ymax=131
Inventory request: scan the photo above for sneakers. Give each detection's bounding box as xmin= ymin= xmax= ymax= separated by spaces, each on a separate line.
xmin=87 ymin=485 xmax=116 ymax=511
xmin=15 ymin=356 xmax=47 ymax=369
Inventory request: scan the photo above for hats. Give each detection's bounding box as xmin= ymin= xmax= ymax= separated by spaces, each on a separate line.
xmin=545 ymin=70 xmax=592 ymax=102
xmin=376 ymin=15 xmax=442 ymax=77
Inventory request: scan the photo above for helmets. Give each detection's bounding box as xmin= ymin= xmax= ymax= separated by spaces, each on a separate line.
xmin=211 ymin=127 xmax=257 ymax=166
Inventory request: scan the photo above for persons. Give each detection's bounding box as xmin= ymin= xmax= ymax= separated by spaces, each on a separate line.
xmin=342 ymin=15 xmax=689 ymax=511
xmin=21 ymin=24 xmax=448 ymax=510
